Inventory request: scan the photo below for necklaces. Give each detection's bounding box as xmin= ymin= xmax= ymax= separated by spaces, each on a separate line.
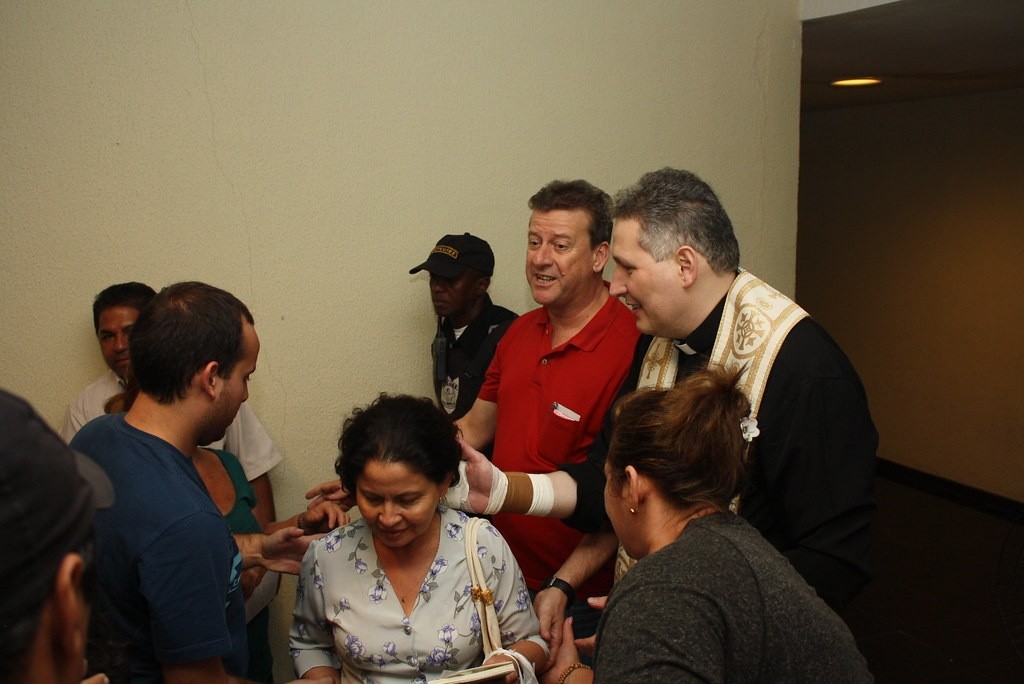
xmin=401 ymin=574 xmax=424 ymax=603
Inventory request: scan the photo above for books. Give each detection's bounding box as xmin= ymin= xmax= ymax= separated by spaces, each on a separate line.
xmin=429 ymin=661 xmax=515 ymax=683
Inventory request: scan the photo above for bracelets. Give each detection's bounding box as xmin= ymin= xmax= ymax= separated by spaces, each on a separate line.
xmin=542 ymin=578 xmax=577 ymax=607
xmin=557 ymin=663 xmax=590 ymax=684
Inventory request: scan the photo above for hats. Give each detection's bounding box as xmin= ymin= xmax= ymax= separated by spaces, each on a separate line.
xmin=0 ymin=389 xmax=94 ymax=585
xmin=409 ymin=232 xmax=495 ymax=277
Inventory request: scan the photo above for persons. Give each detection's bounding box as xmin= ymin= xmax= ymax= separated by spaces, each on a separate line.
xmin=438 ymin=166 xmax=879 ymax=659
xmin=63 ymin=282 xmax=351 ymax=683
xmin=66 ymin=280 xmax=334 ymax=684
xmin=409 ymin=232 xmax=520 ymax=420
xmin=546 ymin=374 xmax=875 ymax=684
xmin=305 ymin=177 xmax=642 ymax=670
xmin=0 ymin=390 xmax=116 ymax=683
xmin=287 ymin=393 xmax=539 ymax=684
